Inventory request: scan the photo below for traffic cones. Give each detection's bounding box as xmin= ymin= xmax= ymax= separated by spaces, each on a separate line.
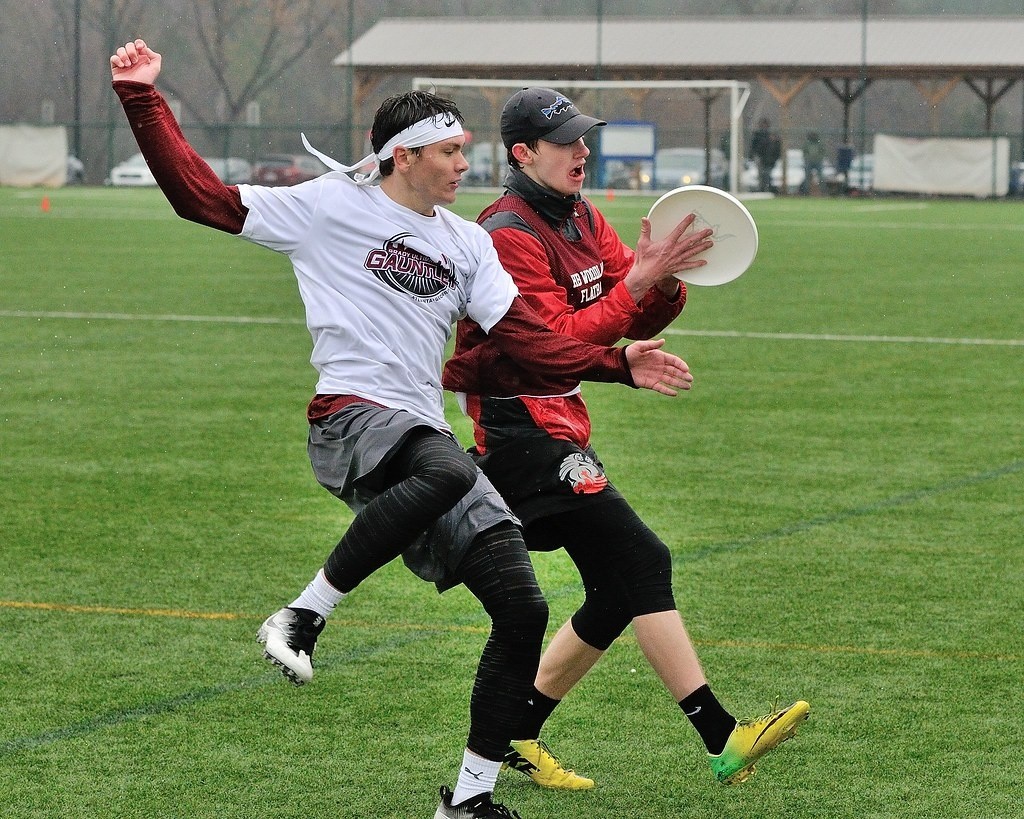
xmin=605 ymin=186 xmax=614 ymax=202
xmin=41 ymin=196 xmax=50 ymax=211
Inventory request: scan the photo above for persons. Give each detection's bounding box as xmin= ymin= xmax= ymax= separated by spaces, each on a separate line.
xmin=108 ymin=37 xmax=694 ymax=819
xmin=716 ymin=116 xmax=854 ymax=199
xmin=448 ymin=87 xmax=810 ymax=793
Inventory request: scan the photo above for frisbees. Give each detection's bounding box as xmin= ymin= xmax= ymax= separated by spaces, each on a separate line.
xmin=647 ymin=183 xmax=759 ymax=286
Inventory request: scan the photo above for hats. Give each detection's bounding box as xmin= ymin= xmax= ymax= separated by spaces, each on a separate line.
xmin=500 ymin=86 xmax=607 ymax=149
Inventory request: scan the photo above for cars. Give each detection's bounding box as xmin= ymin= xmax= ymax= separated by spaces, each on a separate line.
xmin=252 ymin=153 xmax=328 ymax=187
xmin=458 ymin=140 xmax=509 ymax=188
xmin=202 ymin=155 xmax=252 ymax=186
xmin=639 ymin=147 xmax=727 ymax=192
xmin=109 ymin=152 xmax=160 ymax=188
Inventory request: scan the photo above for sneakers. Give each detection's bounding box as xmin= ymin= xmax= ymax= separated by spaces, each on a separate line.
xmin=499 ymin=739 xmax=595 ymax=790
xmin=255 ymin=605 xmax=326 ymax=688
xmin=434 ymin=785 xmax=523 ymax=819
xmin=706 ymin=700 xmax=810 ymax=785
